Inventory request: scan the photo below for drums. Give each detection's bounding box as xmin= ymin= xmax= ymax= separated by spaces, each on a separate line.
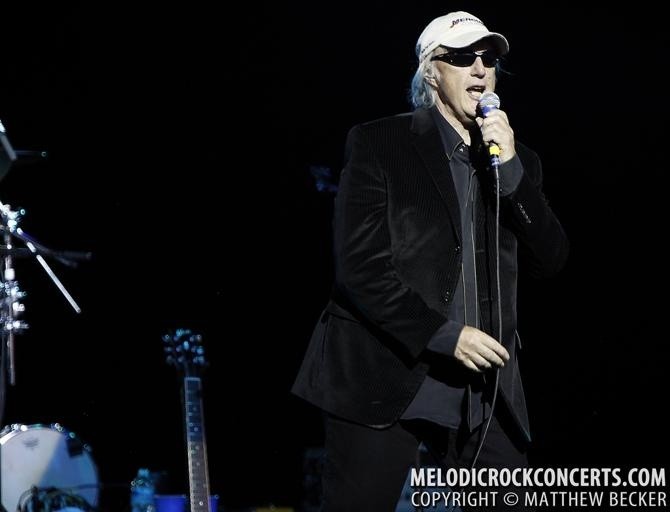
xmin=1 ymin=423 xmax=99 ymax=512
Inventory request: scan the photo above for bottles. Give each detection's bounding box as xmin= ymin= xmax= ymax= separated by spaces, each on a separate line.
xmin=130 ymin=468 xmax=157 ymax=512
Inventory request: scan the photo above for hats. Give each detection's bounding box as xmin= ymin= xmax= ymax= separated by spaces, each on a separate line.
xmin=415 ymin=10 xmax=510 ymax=63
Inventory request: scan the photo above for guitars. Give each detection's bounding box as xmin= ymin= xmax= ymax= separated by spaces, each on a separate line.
xmin=162 ymin=327 xmax=212 ymax=511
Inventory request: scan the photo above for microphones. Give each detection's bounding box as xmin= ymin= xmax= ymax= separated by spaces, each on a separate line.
xmin=478 ymin=92 xmax=501 ymax=168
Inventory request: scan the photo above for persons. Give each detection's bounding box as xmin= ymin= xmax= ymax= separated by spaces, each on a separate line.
xmin=287 ymin=9 xmax=570 ymax=512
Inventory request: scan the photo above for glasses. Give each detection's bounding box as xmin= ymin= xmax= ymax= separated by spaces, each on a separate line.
xmin=430 ymin=50 xmax=502 ymax=68
